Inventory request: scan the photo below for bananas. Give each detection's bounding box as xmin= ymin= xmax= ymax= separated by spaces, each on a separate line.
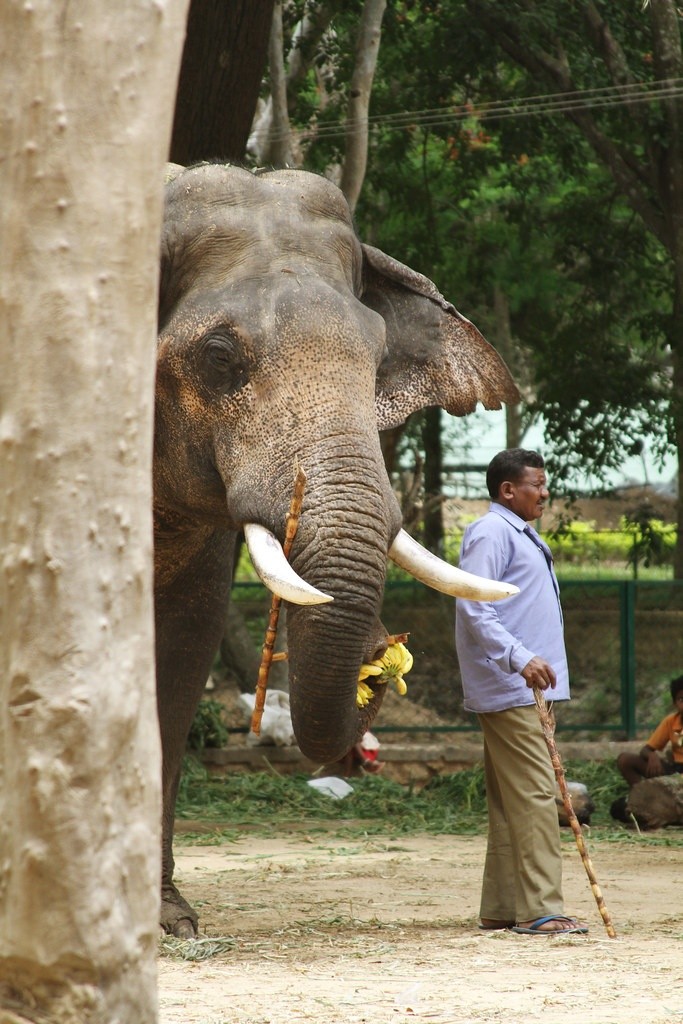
xmin=356 ymin=643 xmax=413 ymax=708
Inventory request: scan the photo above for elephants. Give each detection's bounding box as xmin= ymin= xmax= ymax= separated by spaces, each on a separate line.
xmin=151 ymin=161 xmax=525 ymax=938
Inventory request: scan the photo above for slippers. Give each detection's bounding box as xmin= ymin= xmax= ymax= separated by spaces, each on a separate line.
xmin=479 ymin=925 xmax=517 ymax=929
xmin=513 ymin=915 xmax=589 ymax=934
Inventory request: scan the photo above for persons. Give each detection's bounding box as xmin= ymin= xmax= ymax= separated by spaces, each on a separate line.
xmin=617 ymin=675 xmax=683 ymax=790
xmin=455 ymin=448 xmax=588 ymax=934
